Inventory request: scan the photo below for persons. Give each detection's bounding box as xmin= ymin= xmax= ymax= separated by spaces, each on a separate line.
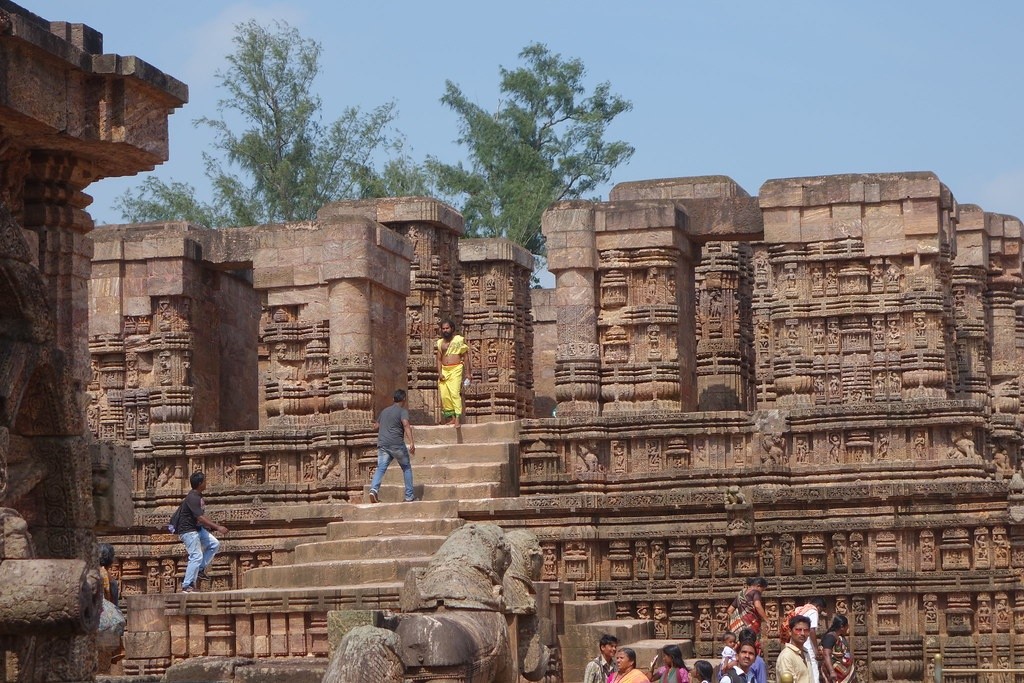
xmin=717 ymin=628 xmax=769 ymax=683
xmin=606 ymin=648 xmax=651 ymax=682
xmin=173 ymin=471 xmax=230 ymax=591
xmin=775 ymin=614 xmax=814 ymax=683
xmin=717 ymin=642 xmax=758 ymax=683
xmin=368 ymin=390 xmax=420 ymax=504
xmin=820 ymin=615 xmax=858 ymax=683
xmin=791 ymin=596 xmax=828 ymax=683
xmin=98 ymin=541 xmax=127 ymax=666
xmin=649 ymin=645 xmax=689 ymax=683
xmin=692 ymin=661 xmax=713 ymax=683
xmin=720 ymin=632 xmax=739 ymax=672
xmin=583 ymin=634 xmax=619 ymax=683
xmin=728 ymin=575 xmax=771 ymax=657
xmin=434 ymin=319 xmax=472 ymax=427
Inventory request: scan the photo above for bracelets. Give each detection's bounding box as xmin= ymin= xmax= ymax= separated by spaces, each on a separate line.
xmin=830 ymin=670 xmax=835 ymax=673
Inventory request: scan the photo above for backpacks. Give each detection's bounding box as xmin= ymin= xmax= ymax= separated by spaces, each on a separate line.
xmin=168 ymin=504 xmax=182 ymax=534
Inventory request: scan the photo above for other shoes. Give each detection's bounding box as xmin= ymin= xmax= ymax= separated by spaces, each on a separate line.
xmin=182 ymin=585 xmax=200 ymax=594
xmin=197 ymin=571 xmax=211 ymax=580
xmin=369 ymin=490 xmax=381 ymax=503
xmin=403 ymin=497 xmax=419 ymax=502
